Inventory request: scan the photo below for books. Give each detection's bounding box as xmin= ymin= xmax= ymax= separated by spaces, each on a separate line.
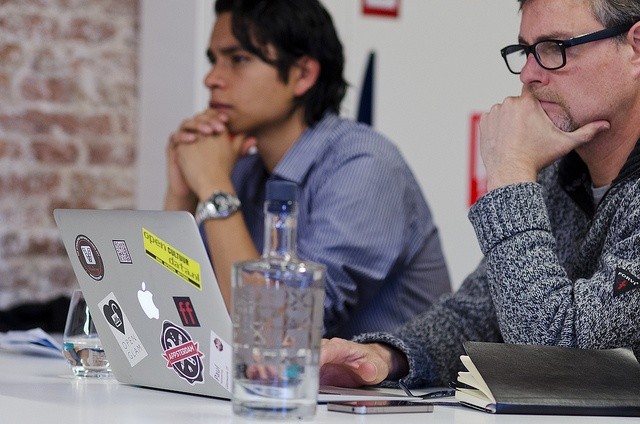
xmin=453 ymin=340 xmax=639 ymax=417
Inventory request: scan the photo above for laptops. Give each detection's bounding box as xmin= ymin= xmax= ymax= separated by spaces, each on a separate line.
xmin=51 ymin=209 xmax=419 ymax=403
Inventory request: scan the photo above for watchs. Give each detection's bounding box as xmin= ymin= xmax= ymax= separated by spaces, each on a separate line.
xmin=192 ymin=189 xmax=247 ymax=232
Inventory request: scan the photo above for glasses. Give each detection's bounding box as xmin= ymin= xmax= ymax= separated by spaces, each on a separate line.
xmin=501 ymin=21 xmax=640 ymax=75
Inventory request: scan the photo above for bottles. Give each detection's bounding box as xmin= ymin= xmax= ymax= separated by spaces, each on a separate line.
xmin=232 ymin=180 xmax=326 ymax=421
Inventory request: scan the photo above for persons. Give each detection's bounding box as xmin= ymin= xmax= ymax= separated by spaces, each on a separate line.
xmin=314 ymin=0 xmax=639 ymax=385
xmin=156 ymin=0 xmax=454 ymax=340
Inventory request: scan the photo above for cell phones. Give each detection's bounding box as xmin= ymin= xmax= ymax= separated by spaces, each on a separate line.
xmin=325 ymin=398 xmax=434 ymax=414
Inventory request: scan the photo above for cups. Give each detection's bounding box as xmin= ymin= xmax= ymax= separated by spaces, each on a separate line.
xmin=64 ymin=289 xmax=110 ymax=377
